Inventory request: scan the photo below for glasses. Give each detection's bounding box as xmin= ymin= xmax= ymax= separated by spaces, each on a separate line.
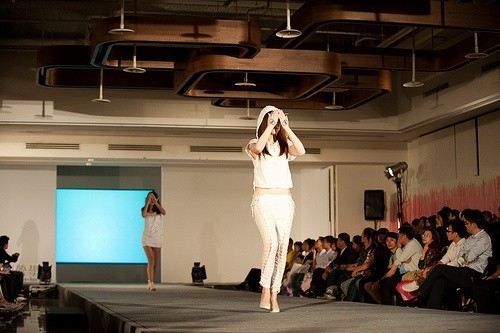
xmin=464 ymin=221 xmax=471 ymax=225
xmin=421 ymin=234 xmax=431 ymax=237
xmin=445 ymin=228 xmax=453 ymax=232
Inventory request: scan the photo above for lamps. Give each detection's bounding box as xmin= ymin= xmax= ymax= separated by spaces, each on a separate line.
xmin=235 ymin=72 xmax=256 ymax=87
xmin=91 ymin=69 xmax=112 ymax=104
xmin=464 ymin=31 xmax=488 ymax=58
xmin=239 ymin=100 xmax=257 ymax=120
xmin=109 ymin=0 xmax=135 ymax=35
xmin=123 ymin=43 xmax=146 ymax=73
xmin=38 ymin=262 xmax=52 ymax=284
xmin=276 ymin=0 xmax=302 ymax=38
xmin=191 ymin=262 xmax=207 ymax=283
xmin=388 ymin=161 xmax=408 ymax=177
xmin=325 ymin=92 xmax=343 ymax=109
xmin=403 ymin=27 xmax=424 ymax=87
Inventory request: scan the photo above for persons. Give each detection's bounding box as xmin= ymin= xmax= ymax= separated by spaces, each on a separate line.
xmin=141 ymin=192 xmax=166 ymax=291
xmin=0 ymin=235 xmax=27 ymax=327
xmin=234 ymin=207 xmax=500 ymax=312
xmin=245 ymin=105 xmax=305 ymax=313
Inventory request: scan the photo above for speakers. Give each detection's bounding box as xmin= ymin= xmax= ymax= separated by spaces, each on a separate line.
xmin=240 ymin=267 xmax=262 ymax=292
xmin=364 ymin=190 xmax=385 ymax=220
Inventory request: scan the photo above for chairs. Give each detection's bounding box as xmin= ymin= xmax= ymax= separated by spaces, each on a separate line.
xmin=480 ymin=251 xmax=497 ymax=279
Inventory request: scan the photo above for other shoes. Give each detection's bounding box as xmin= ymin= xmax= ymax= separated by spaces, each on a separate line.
xmin=323 ymin=292 xmax=336 ymax=300
xmin=299 ymin=291 xmax=317 ymax=298
xmin=269 ymin=306 xmax=280 ymax=313
xmin=259 ymin=294 xmax=271 ymax=310
xmin=147 ymin=280 xmax=156 ymax=291
